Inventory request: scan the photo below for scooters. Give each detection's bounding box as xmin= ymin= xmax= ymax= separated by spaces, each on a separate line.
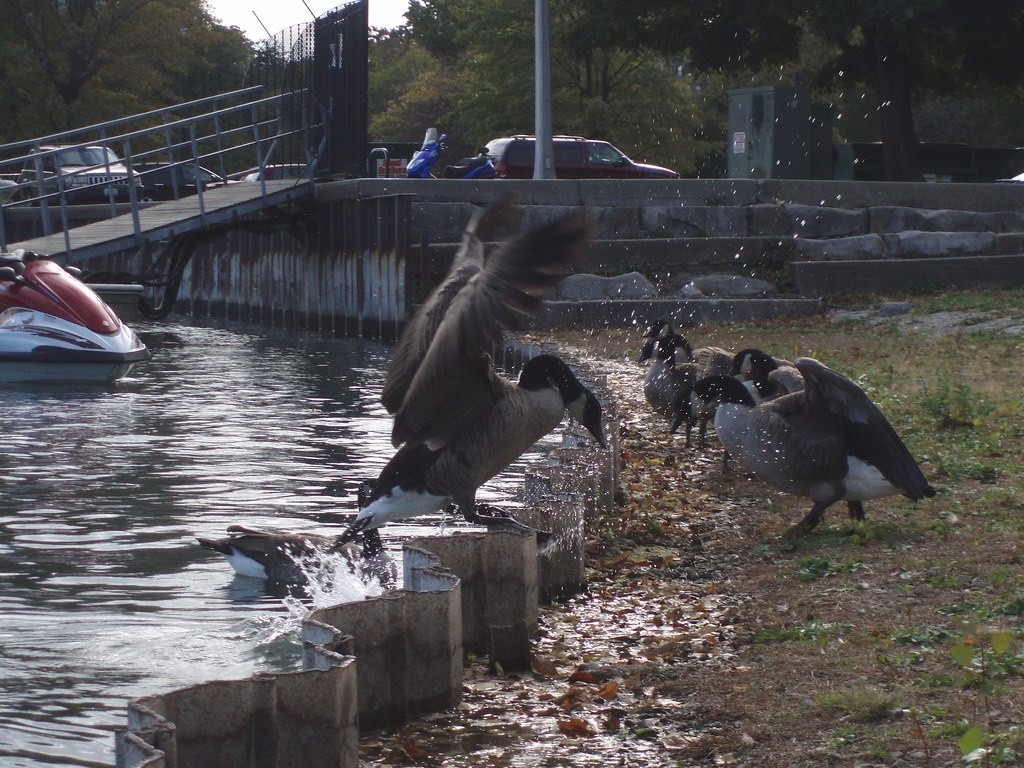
xmin=407 ymin=127 xmax=500 ymax=179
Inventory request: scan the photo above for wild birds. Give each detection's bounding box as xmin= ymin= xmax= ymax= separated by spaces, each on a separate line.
xmin=635 ymin=318 xmax=938 ymax=555
xmin=193 ymin=188 xmax=610 ymax=590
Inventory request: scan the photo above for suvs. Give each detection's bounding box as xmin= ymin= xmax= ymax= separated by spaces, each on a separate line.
xmin=476 ymin=134 xmax=678 ymax=180
xmin=20 ymin=144 xmax=145 ymax=204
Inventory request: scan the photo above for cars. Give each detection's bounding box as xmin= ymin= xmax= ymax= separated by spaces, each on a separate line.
xmin=127 ymin=161 xmax=242 ymax=200
xmin=221 ymin=164 xmax=312 ymax=183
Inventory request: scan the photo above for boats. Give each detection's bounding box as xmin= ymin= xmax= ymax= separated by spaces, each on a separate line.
xmin=0 ymin=248 xmax=155 ymax=386
